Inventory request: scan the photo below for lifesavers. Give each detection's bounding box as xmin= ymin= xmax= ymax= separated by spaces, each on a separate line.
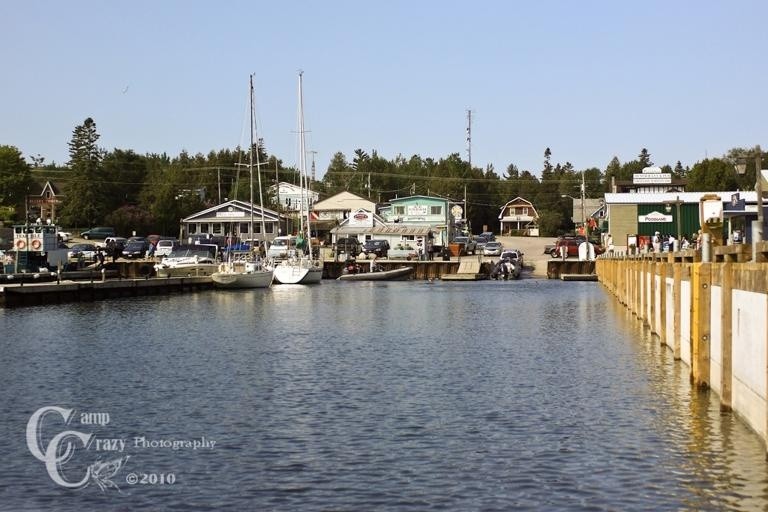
xmin=16 ymin=239 xmax=25 ymax=249
xmin=32 ymin=239 xmax=41 ymax=249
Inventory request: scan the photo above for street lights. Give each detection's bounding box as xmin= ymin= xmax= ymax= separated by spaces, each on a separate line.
xmin=561 ymin=194 xmax=584 ymax=228
xmin=661 ymin=195 xmax=684 ymax=248
xmin=728 ymin=142 xmax=766 ymax=245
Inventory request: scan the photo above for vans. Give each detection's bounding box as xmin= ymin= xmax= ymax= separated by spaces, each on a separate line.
xmin=77 ymin=226 xmax=117 ymax=241
xmin=361 ymin=239 xmax=390 ymax=258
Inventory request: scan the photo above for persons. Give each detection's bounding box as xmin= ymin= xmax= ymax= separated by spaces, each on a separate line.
xmin=653 ymin=228 xmax=742 ymax=254
xmin=147 ymin=240 xmax=157 ymax=258
xmin=427 ymin=240 xmax=434 ymax=261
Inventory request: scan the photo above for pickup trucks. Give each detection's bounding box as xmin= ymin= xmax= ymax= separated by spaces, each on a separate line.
xmin=331 ymin=236 xmax=363 ymax=257
xmin=452 ymin=236 xmax=477 ymax=255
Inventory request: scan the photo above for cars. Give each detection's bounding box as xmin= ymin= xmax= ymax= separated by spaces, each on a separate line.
xmin=471 ymin=235 xmax=504 ymax=256
xmin=66 ymin=233 xmax=180 ymax=263
xmin=54 ymin=229 xmax=75 ymax=242
xmin=185 ymin=232 xmax=299 ymax=260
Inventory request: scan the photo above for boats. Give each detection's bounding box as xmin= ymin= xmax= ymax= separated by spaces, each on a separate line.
xmin=0 ymin=218 xmax=120 ymax=280
xmin=336 ymin=264 xmax=417 ymax=283
xmin=490 ymin=257 xmax=522 ymax=280
xmin=151 ymin=242 xmax=224 ymax=280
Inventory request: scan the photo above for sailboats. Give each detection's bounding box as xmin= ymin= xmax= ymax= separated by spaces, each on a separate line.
xmin=210 ymin=70 xmax=279 ymax=290
xmin=263 ymin=68 xmax=324 ymax=287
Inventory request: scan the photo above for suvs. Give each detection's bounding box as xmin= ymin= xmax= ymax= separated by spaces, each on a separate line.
xmin=478 ymin=231 xmax=494 ymax=242
xmin=542 ymin=233 xmax=602 ymax=259
xmin=500 ymin=248 xmax=524 ymax=269
